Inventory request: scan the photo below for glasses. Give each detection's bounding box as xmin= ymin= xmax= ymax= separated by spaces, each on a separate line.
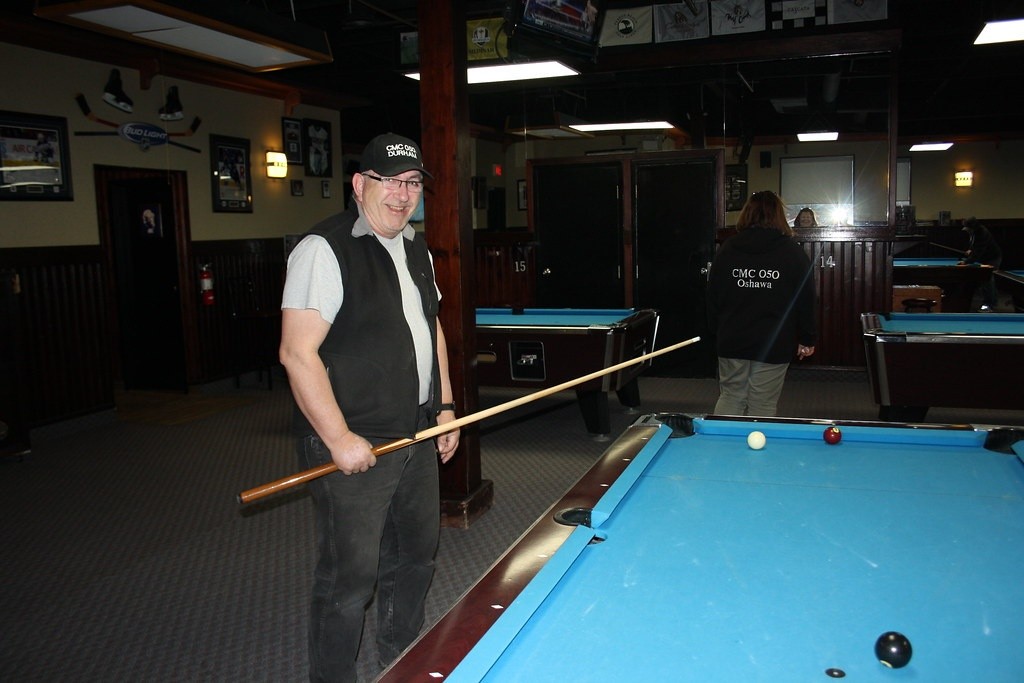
xmin=361 ymin=172 xmax=425 ymax=193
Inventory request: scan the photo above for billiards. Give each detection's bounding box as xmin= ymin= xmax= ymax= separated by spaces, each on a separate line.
xmin=748 ymin=431 xmax=766 ymax=449
xmin=824 ymin=427 xmax=841 ymax=444
xmin=876 ymin=631 xmax=912 ymax=668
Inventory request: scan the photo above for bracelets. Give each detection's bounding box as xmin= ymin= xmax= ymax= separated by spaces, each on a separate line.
xmin=442 ymin=401 xmax=456 ymax=411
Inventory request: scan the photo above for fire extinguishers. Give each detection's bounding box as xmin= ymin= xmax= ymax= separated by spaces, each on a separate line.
xmin=200 ymin=261 xmax=215 ymax=306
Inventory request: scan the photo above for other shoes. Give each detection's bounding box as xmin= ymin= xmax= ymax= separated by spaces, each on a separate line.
xmin=980 ymin=304 xmax=992 ymax=312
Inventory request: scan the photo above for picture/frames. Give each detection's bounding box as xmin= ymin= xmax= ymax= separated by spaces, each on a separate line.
xmin=517 ymin=180 xmax=528 ymax=211
xmin=208 ymin=135 xmax=253 ymax=212
xmin=0 ymin=109 xmax=74 ymax=202
xmin=726 ymin=163 xmax=748 ymax=212
xmin=281 ymin=117 xmax=304 ymax=165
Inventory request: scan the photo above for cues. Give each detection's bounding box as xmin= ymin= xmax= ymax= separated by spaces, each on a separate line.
xmin=930 ymin=242 xmax=966 ymax=253
xmin=239 ymin=336 xmax=701 ymax=505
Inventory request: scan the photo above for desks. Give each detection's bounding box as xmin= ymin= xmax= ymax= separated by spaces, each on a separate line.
xmin=474 ymin=307 xmax=657 ymax=444
xmin=991 ymin=268 xmax=1024 ymax=313
xmin=858 ymin=308 xmax=1024 ymax=412
xmin=890 ymin=258 xmax=994 ymax=309
xmin=367 ymin=411 xmax=1024 ymax=683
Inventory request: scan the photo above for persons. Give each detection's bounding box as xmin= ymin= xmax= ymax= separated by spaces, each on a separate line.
xmin=957 ymin=216 xmax=1002 ymax=313
xmin=279 ymin=133 xmax=461 ymax=683
xmin=794 ymin=207 xmax=818 ymax=227
xmin=705 ymin=191 xmax=816 ymax=417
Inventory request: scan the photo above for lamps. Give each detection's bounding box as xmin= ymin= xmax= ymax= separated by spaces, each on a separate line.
xmin=265 ymin=150 xmax=288 ymax=177
xmin=796 ymin=111 xmax=838 ymax=142
xmin=954 ymin=171 xmax=974 ymax=187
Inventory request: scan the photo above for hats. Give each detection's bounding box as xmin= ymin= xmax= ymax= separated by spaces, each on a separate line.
xmin=962 ymin=215 xmax=976 ymax=231
xmin=359 ymin=133 xmax=433 ymax=181
